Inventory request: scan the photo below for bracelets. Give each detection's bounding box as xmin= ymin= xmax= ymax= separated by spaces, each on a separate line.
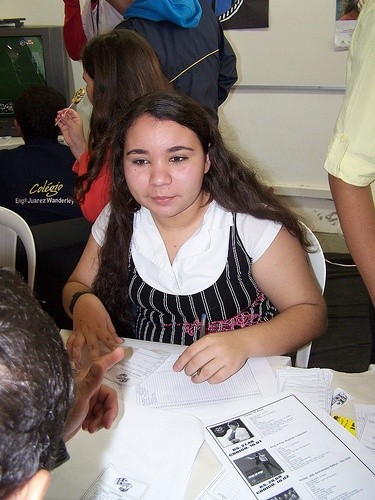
xmin=68 ymin=289 xmax=98 ymax=315
xmin=39 ymin=427 xmax=72 ymax=474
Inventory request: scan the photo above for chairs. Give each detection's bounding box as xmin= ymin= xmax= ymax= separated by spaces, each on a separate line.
xmin=0 ymin=204 xmax=37 ymax=297
xmin=290 ymin=219 xmax=328 ymax=368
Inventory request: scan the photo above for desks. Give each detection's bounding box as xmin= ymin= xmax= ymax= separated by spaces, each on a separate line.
xmin=47 ymin=329 xmax=375 ymax=500
xmin=0 ymin=136 xmax=68 ymax=150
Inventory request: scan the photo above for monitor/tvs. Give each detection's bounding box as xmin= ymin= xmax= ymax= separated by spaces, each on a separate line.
xmin=0 ymin=26 xmax=75 ymax=136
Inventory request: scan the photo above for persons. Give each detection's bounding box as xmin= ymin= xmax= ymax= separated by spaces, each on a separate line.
xmin=62 ymin=0 xmax=126 ymax=62
xmin=55 ymin=29 xmax=176 ymax=223
xmin=227 ymin=420 xmax=251 ymax=443
xmin=321 ymin=1 xmax=375 ymax=310
xmin=107 ymin=1 xmax=239 ymax=113
xmin=0 ymin=266 xmax=124 ymax=500
xmin=244 ymin=452 xmax=284 ymax=478
xmin=0 ymin=82 xmax=85 ymax=229
xmin=268 ymin=487 xmax=302 ymax=500
xmin=62 ymin=89 xmax=328 ymax=386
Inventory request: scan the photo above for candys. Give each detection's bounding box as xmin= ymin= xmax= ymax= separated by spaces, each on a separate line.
xmin=72 ymin=88 xmax=84 ymax=105
